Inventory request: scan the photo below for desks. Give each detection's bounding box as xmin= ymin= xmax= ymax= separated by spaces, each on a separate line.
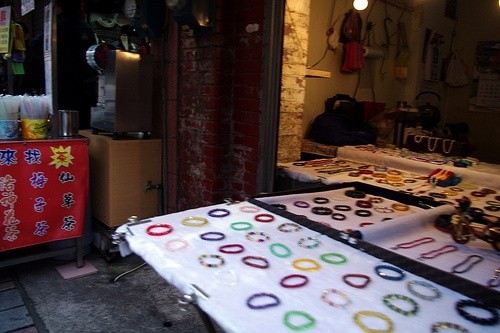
xmin=78 ymin=129 xmax=162 ymax=232
xmin=111 ymin=184 xmax=498 ymax=333
xmin=1 ymin=137 xmax=91 ymax=273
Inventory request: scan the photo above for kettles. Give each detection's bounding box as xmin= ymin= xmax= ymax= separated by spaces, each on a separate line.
xmin=414 ymin=91 xmax=443 ymax=127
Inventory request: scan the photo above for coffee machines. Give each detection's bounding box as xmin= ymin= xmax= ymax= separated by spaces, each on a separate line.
xmin=86 ymin=42 xmax=157 ymax=140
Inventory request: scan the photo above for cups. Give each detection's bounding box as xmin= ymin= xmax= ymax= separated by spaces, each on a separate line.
xmin=397 ymin=101 xmax=411 ymax=111
xmin=57 ymin=110 xmax=80 ymax=136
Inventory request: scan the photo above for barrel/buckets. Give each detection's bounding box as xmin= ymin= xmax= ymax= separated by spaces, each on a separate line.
xmin=0 ymin=112 xmax=21 ymax=139
xmin=20 ymin=113 xmax=51 ymax=140
